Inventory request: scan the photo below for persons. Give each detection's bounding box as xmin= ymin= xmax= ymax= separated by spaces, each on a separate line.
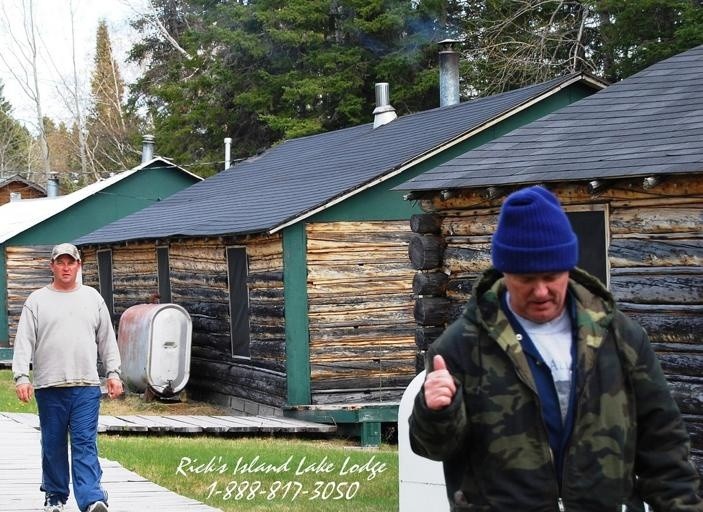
xmin=10 ymin=242 xmax=123 ymax=511
xmin=406 ymin=187 xmax=701 ymax=510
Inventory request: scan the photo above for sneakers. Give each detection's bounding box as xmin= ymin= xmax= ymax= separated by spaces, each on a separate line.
xmin=86 ymin=501 xmax=108 ymax=512
xmin=43 ymin=493 xmax=64 ymax=512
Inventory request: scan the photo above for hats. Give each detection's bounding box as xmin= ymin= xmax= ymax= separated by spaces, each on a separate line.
xmin=51 ymin=242 xmax=81 ymax=263
xmin=490 ymin=186 xmax=579 ymax=272
